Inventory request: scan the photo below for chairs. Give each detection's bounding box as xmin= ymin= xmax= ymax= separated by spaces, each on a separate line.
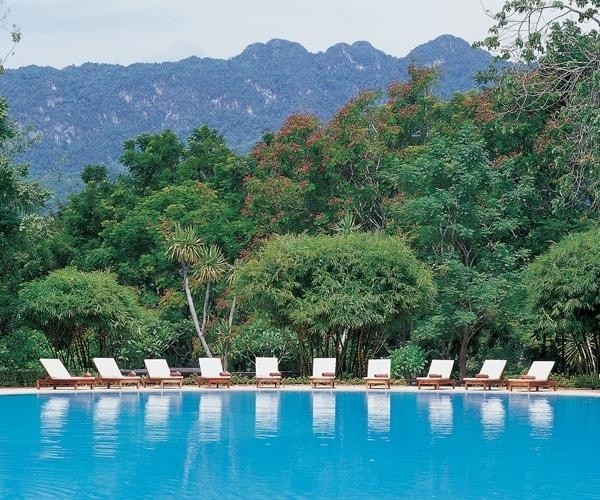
xmin=92 ymin=358 xmax=141 ymax=389
xmin=363 ymin=359 xmax=392 ymax=389
xmin=506 ymin=361 xmax=557 ymax=391
xmin=308 ymin=358 xmax=337 ymax=388
xmin=255 ymin=357 xmax=282 ymax=388
xmin=462 ymin=360 xmax=507 ymax=391
xmin=36 ymin=358 xmax=96 ymax=389
xmin=416 ymin=359 xmax=455 ymax=390
xmin=142 ymin=359 xmax=184 ymax=389
xmin=197 ymin=357 xmax=232 ymax=388
